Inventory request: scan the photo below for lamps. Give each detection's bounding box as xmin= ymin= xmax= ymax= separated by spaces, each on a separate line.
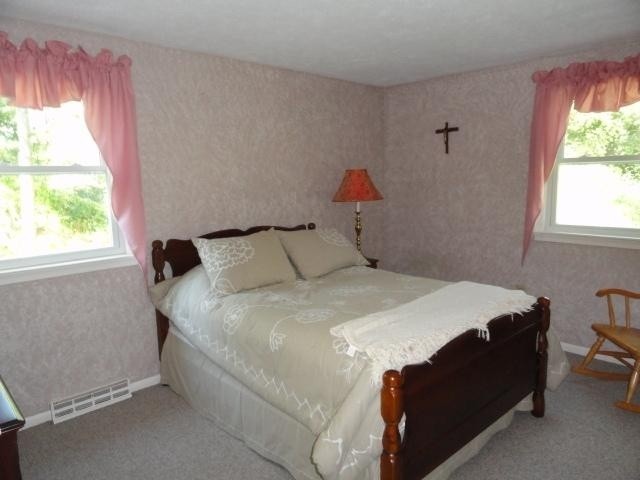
xmin=331 ymin=168 xmax=384 ymax=259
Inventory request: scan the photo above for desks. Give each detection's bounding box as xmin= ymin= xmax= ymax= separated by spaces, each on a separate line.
xmin=0 ymin=373 xmax=25 ymax=480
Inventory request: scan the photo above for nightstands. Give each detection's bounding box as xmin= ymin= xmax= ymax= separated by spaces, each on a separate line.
xmin=364 ymin=255 xmax=379 ymax=269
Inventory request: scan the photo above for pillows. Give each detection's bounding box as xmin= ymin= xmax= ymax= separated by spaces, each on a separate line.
xmin=274 ymin=226 xmax=372 ymax=281
xmin=190 ymin=229 xmax=297 ymax=299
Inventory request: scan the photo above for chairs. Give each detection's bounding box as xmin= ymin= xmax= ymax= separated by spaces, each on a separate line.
xmin=571 ymin=287 xmax=640 ymax=414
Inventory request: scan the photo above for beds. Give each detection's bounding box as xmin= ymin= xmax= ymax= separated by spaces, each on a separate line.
xmin=148 ymin=223 xmax=551 ymax=480
